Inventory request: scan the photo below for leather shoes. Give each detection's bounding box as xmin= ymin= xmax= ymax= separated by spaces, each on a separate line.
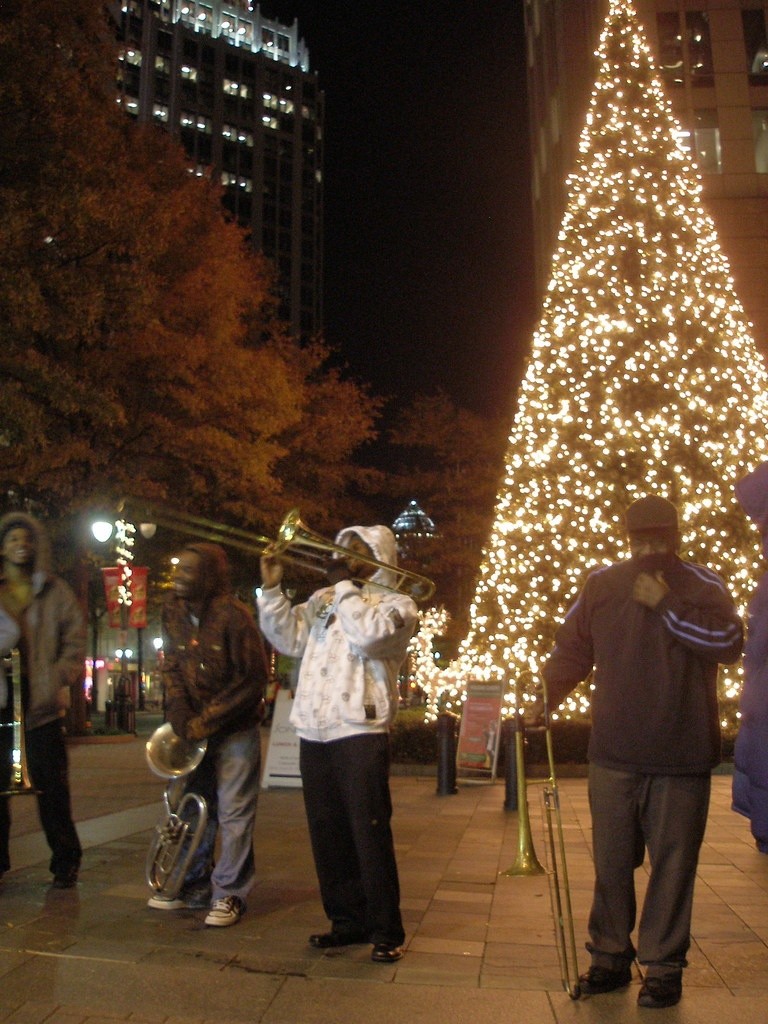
xmin=371 ymin=943 xmax=402 ymax=962
xmin=308 ymin=931 xmax=369 ymax=947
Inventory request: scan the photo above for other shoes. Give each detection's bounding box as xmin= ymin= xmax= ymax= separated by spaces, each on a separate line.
xmin=637 ymin=978 xmax=682 ymax=1008
xmin=54 ymin=858 xmax=80 ymax=887
xmin=577 ymin=966 xmax=628 ymax=993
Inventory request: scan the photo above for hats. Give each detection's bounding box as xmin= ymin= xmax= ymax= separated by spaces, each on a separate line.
xmin=625 ymin=494 xmax=679 ymax=535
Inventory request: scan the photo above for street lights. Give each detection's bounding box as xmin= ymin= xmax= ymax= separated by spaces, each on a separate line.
xmin=91 ymin=509 xmax=157 ymax=733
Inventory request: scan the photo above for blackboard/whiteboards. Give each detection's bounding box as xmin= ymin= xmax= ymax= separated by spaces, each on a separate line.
xmin=263 ymin=689 xmax=301 ymax=775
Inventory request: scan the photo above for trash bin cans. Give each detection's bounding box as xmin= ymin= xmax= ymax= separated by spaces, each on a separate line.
xmin=104 ymin=699 xmax=121 ymax=733
xmin=121 ymin=698 xmax=136 ymax=733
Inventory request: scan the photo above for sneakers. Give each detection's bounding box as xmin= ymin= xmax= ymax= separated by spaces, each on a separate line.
xmin=148 ymin=894 xmax=206 ymax=909
xmin=205 ymin=896 xmax=242 ymax=926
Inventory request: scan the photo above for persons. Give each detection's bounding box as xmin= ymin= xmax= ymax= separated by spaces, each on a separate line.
xmin=0 ymin=513 xmax=94 ymax=889
xmin=524 ymin=495 xmax=743 ymax=1008
xmin=254 ymin=524 xmax=419 ymax=963
xmin=737 ymin=456 xmax=768 ymax=860
xmin=146 ymin=539 xmax=272 ymax=927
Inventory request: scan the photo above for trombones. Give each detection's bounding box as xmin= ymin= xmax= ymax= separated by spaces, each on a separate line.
xmin=1 ymin=645 xmax=47 ymax=797
xmin=115 ymin=490 xmax=438 ymax=606
xmin=492 ymin=670 xmax=583 ymax=1000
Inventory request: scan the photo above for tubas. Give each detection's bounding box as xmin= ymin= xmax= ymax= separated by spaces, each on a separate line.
xmin=138 ymin=719 xmax=211 ymax=902
